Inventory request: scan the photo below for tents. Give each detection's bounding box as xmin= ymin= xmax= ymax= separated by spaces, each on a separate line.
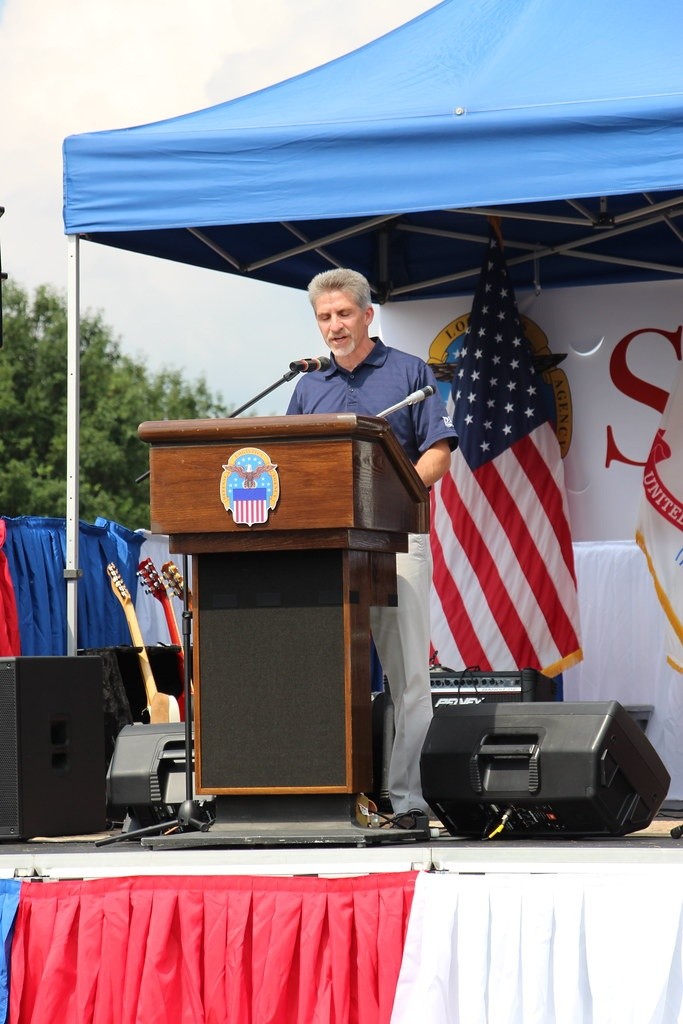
xmin=62 ymin=1 xmax=683 ymax=656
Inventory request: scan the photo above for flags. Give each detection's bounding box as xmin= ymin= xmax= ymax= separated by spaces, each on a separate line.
xmin=634 ymin=359 xmax=683 ymax=673
xmin=428 ymin=228 xmax=585 ymax=679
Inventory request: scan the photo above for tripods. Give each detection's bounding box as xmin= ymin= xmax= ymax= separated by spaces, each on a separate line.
xmin=92 ymin=371 xmax=296 ymax=848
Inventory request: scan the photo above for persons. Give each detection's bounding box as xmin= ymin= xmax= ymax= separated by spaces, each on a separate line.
xmin=283 ymin=267 xmax=458 ymax=822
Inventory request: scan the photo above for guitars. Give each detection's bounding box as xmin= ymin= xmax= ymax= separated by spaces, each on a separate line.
xmin=139 ymin=559 xmax=188 ymax=721
xmin=159 ymin=561 xmax=195 ymax=619
xmin=106 ymin=561 xmax=179 ymax=723
xmin=137 ymin=559 xmax=187 ymax=722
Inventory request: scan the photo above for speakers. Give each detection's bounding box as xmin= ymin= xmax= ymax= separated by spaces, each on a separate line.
xmin=0 ymin=655 xmax=104 ymax=838
xmin=105 ymin=720 xmax=216 ymax=837
xmin=428 ymin=671 xmax=556 ymax=717
xmin=419 ymin=699 xmax=671 ymax=839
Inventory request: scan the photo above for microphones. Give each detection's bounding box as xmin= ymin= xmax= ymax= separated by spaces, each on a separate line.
xmin=289 ymin=356 xmax=331 ymax=372
xmin=376 ymin=385 xmax=437 ymax=418
